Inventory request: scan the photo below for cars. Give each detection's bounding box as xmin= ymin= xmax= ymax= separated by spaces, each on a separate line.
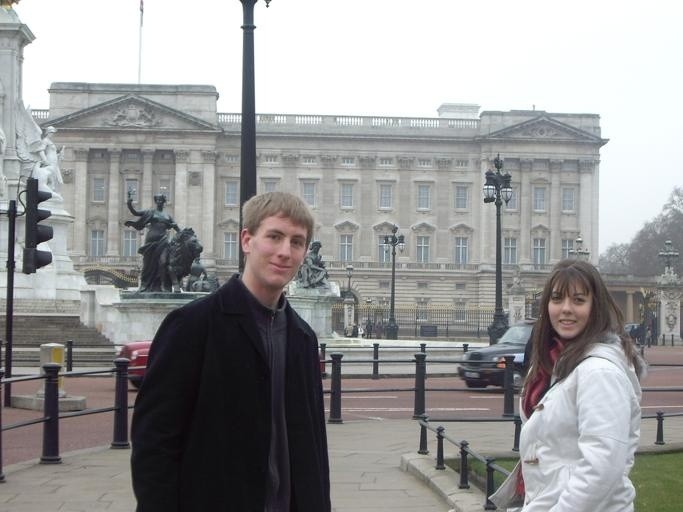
xmin=623 ymin=322 xmax=639 ymax=335
xmin=118 ymin=336 xmax=328 ymax=389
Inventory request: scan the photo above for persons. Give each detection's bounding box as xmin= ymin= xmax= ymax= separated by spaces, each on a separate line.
xmin=483 ymin=256 xmax=648 ymax=511
xmin=126 ymin=190 xmax=179 ymax=289
xmin=344 ymin=319 xmax=384 ymax=339
xmin=290 ymin=240 xmax=329 ymax=287
xmin=629 ymin=323 xmax=654 ymax=348
xmin=126 ymin=189 xmax=334 ymax=509
xmin=33 ymin=124 xmax=66 ymax=193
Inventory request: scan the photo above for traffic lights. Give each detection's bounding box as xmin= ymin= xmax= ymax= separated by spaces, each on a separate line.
xmin=23 ymin=175 xmax=56 ymax=278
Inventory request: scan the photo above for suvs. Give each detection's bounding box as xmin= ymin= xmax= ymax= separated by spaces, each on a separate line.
xmin=458 ymin=322 xmax=541 ymax=390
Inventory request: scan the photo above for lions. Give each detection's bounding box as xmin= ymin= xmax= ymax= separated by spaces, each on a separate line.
xmin=139 ymin=226 xmax=204 ymax=294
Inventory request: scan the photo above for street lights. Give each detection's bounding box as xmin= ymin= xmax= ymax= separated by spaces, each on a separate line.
xmin=344 ymin=263 xmax=355 ymax=294
xmin=655 ymin=239 xmax=682 ymax=284
xmin=482 ymin=149 xmax=511 ymax=345
xmin=382 ymin=223 xmax=407 ymax=337
